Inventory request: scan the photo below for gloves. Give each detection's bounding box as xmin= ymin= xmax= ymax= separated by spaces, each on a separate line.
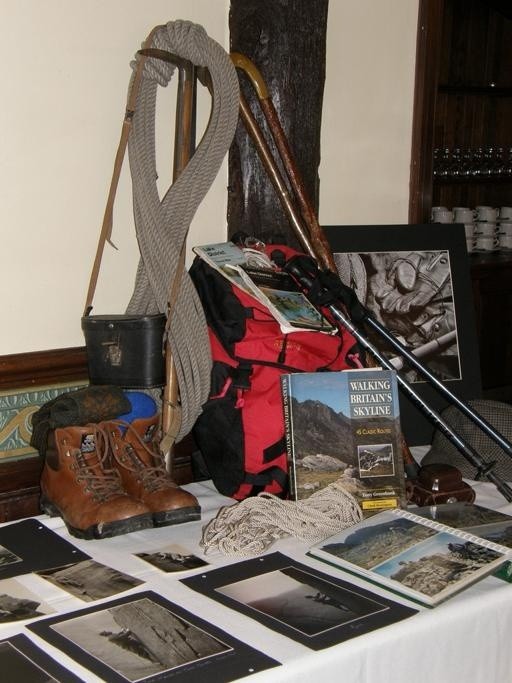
xmin=29 ymin=385 xmax=132 ymax=456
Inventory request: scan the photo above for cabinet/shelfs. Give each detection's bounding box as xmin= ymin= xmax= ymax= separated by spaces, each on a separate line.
xmin=408 ymin=119 xmax=512 ymax=402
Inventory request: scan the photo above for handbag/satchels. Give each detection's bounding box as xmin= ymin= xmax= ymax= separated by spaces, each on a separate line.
xmin=81 ymin=312 xmax=168 ymax=390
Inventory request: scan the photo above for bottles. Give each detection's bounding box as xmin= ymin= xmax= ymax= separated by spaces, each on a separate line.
xmin=432 ymin=147 xmax=511 ymax=176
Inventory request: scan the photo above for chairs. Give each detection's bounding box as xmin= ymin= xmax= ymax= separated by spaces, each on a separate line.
xmin=424 ymin=400 xmax=512 ymax=482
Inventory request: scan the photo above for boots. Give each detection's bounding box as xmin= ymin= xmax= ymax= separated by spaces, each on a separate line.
xmin=39 ymin=423 xmax=154 ymax=540
xmin=102 ymin=390 xmax=200 ymax=527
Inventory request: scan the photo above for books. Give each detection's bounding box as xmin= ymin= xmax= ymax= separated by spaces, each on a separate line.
xmin=303 ymin=500 xmax=512 ymax=609
xmin=193 ymin=240 xmax=266 ymax=302
xmin=234 ymin=256 xmax=331 ymax=337
xmin=279 ymin=363 xmax=405 ymax=522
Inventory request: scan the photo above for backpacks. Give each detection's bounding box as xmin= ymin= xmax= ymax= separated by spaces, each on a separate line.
xmin=188 ymin=245 xmax=371 ymax=502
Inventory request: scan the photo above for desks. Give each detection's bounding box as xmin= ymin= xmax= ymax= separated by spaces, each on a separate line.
xmin=0 ymin=442 xmax=512 ymax=681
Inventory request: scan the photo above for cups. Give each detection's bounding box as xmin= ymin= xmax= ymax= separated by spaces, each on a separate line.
xmin=428 ymin=206 xmax=511 ymax=258
